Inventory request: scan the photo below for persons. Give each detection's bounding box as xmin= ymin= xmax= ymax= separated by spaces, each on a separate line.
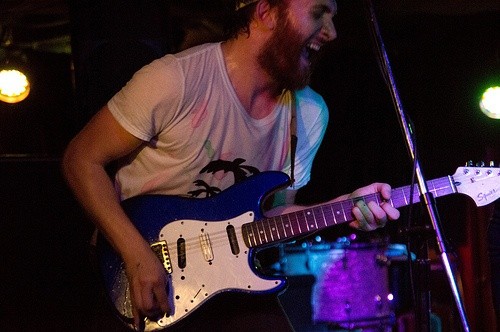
xmin=22 ymin=38 xmax=57 ymax=81
xmin=61 ymin=0 xmax=400 ymax=332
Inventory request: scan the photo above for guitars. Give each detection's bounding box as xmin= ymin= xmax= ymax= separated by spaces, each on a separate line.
xmin=97 ymin=160 xmax=500 ymax=332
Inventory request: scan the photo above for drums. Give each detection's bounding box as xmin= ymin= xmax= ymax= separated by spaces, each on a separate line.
xmin=274 ymin=241 xmax=408 ymax=332
